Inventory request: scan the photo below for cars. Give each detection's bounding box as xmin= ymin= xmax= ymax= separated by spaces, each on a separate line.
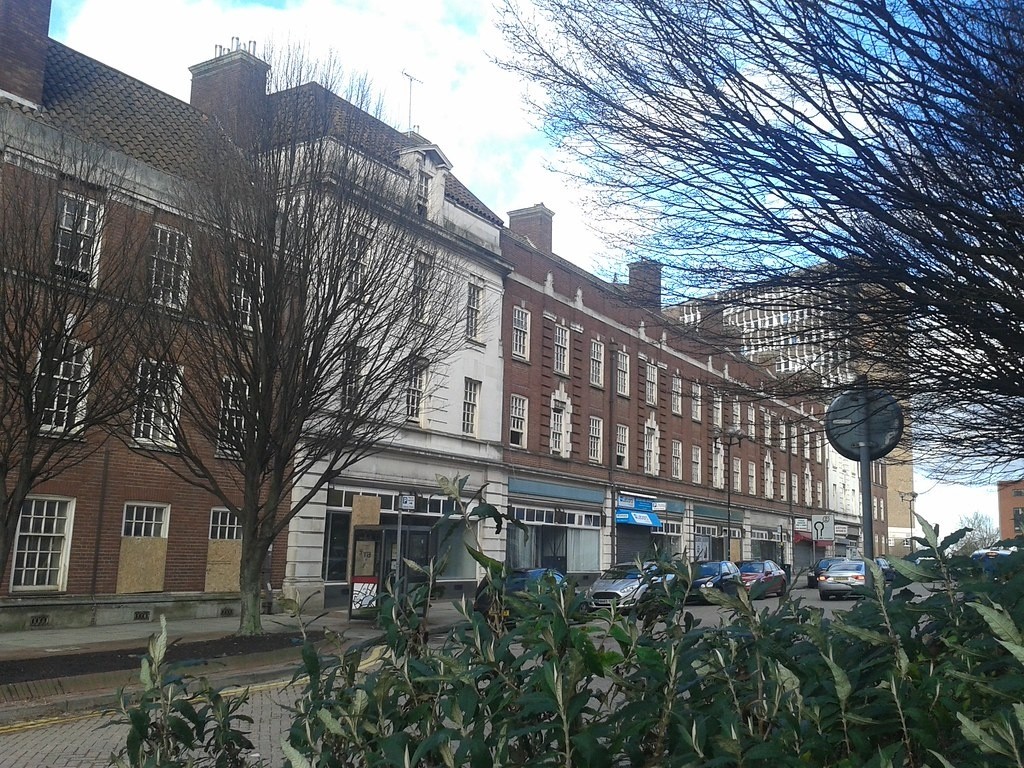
xmin=589 ymin=563 xmax=680 ymax=615
xmin=817 ymin=562 xmax=867 ymax=601
xmin=806 ymin=557 xmax=850 ymax=587
xmin=475 ymin=569 xmax=591 ymax=624
xmin=729 ymin=560 xmax=787 ymax=598
xmin=969 ymin=550 xmax=1013 ymax=567
xmin=849 ymin=558 xmax=896 ymax=583
xmin=679 ymin=561 xmax=741 ymax=603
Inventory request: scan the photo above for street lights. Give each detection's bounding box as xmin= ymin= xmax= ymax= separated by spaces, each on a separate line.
xmin=900 ymin=491 xmax=917 ymax=553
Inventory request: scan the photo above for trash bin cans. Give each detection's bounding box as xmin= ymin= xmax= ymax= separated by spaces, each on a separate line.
xmin=780 ymin=563 xmax=792 ymax=585
